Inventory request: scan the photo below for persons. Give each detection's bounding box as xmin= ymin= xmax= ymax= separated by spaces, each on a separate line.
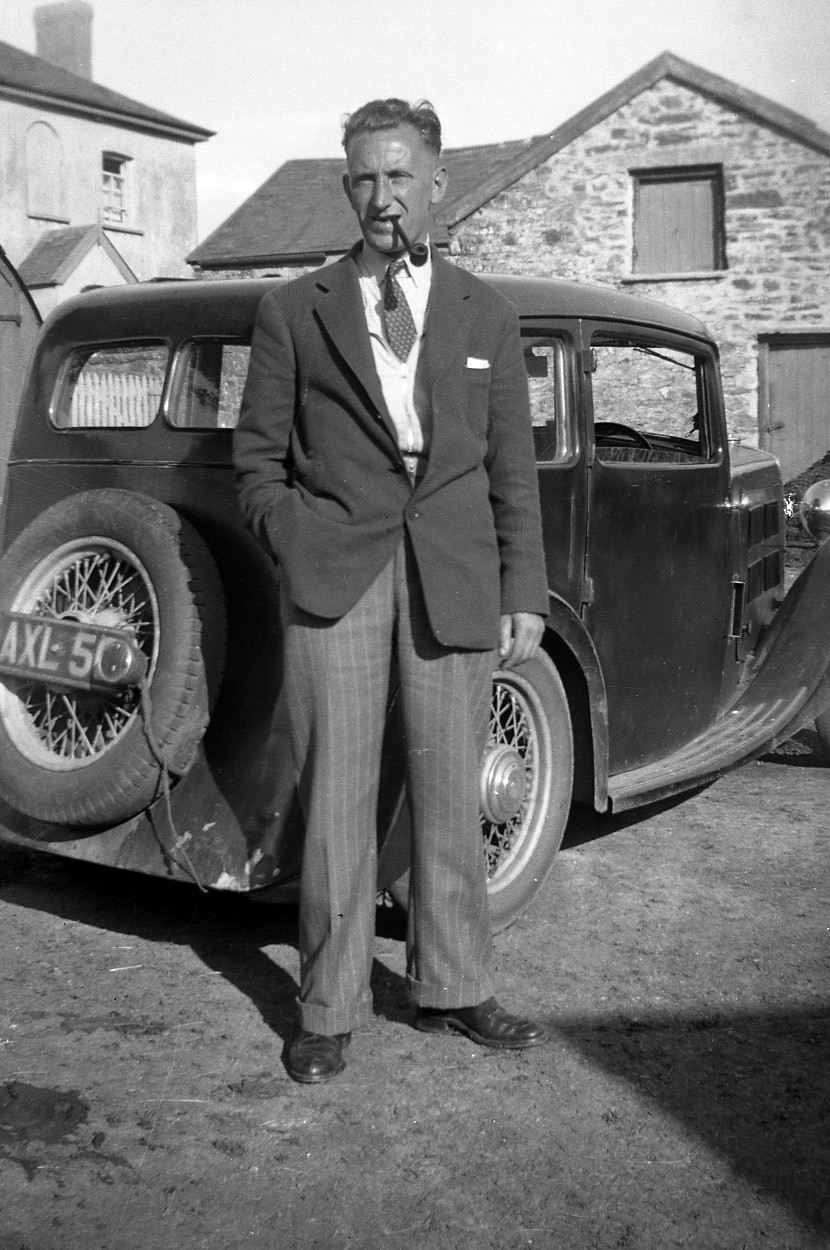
xmin=231 ymin=98 xmax=550 ymax=1083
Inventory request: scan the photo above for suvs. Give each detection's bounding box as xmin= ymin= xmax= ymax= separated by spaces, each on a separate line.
xmin=1 ymin=272 xmax=830 ymax=941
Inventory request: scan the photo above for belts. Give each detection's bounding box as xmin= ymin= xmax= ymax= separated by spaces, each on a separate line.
xmin=403 ymin=456 xmax=429 ymax=477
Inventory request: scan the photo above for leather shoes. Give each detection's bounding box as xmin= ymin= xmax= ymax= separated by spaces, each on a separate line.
xmin=289 ymin=1027 xmax=352 ymax=1082
xmin=414 ymin=997 xmax=547 ymax=1048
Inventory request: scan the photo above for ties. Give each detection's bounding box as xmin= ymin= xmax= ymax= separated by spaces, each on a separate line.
xmin=383 ymin=260 xmax=417 ymax=363
